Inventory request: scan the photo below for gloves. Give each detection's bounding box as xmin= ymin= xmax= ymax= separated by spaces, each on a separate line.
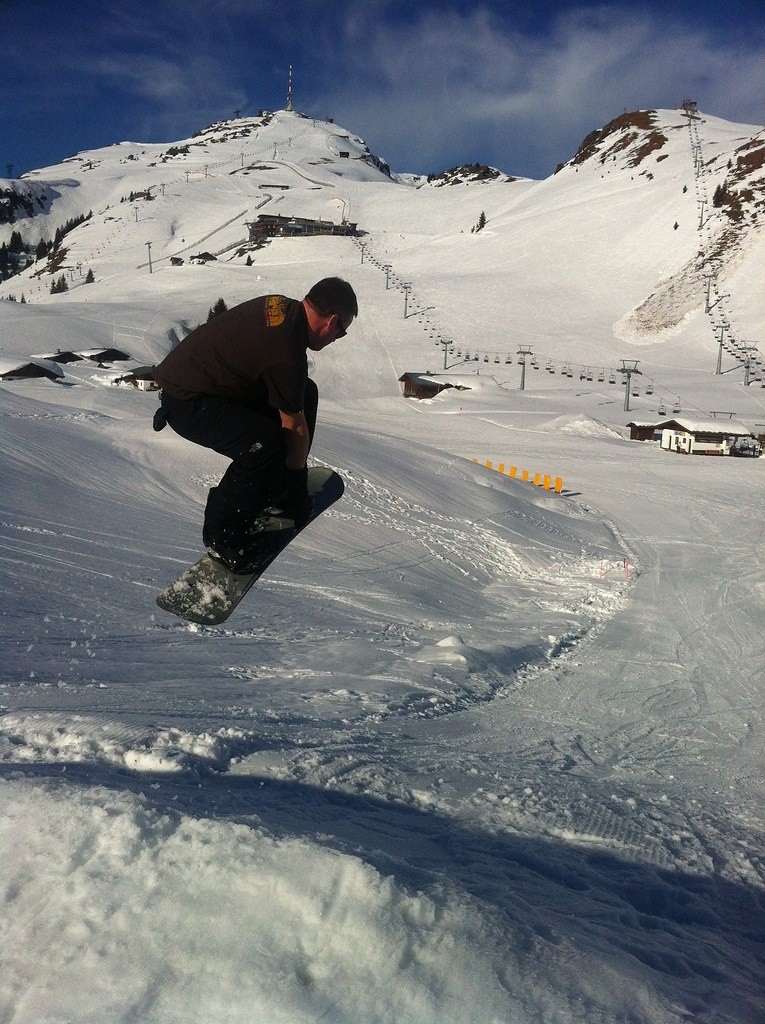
xmin=289 ymin=463 xmax=310 ymax=498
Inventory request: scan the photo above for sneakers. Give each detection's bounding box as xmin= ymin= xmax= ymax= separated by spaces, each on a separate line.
xmin=262 ymin=497 xmax=313 ymax=518
xmin=206 ymin=545 xmax=259 ymax=574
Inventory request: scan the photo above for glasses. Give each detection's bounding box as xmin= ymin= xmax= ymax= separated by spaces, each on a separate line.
xmin=336 ymin=319 xmax=347 ymax=339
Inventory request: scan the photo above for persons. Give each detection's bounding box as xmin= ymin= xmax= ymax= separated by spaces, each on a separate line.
xmin=149 ymin=276 xmax=359 ymax=575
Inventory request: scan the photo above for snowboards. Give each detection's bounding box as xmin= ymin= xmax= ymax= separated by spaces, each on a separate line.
xmin=153 ymin=465 xmax=349 ymax=626
